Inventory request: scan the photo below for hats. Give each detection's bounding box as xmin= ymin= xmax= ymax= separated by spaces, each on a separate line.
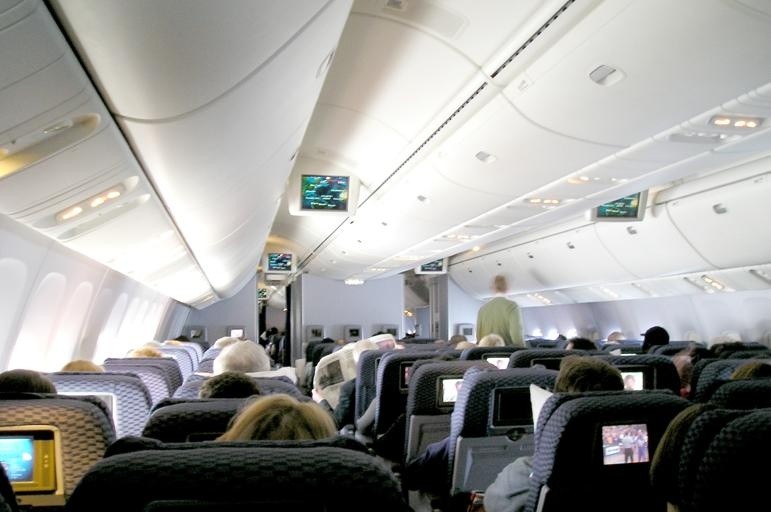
xmin=641 ymin=326 xmax=669 ymax=345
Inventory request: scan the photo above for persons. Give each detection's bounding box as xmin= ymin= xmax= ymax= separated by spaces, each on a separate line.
xmin=476 ymin=275 xmax=526 ymax=348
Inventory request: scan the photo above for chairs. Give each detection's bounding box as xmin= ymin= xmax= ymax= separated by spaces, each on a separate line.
xmin=0 ymin=335 xmax=770 ymax=511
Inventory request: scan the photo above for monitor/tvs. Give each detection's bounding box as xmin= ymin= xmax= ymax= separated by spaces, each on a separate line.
xmin=615 ymin=363 xmax=658 ymax=391
xmin=306 ymin=324 xmax=476 ymax=345
xmin=600 ymin=418 xmax=653 ymax=471
xmin=265 ymin=251 xmax=297 ymax=273
xmin=373 ymin=356 xmax=380 ymax=390
xmin=258 ymin=285 xmax=270 ymax=300
xmin=436 ymin=374 xmax=471 ymax=412
xmin=490 ymin=385 xmax=549 ymax=431
xmin=532 ymin=356 xmax=567 ymax=371
xmin=590 ymin=189 xmax=648 ymax=221
xmin=415 ymin=257 xmax=449 ymax=274
xmin=397 ymin=359 xmax=413 ymax=396
xmin=287 ymin=166 xmax=359 ymax=218
xmin=0 ymin=426 xmax=63 ymax=501
xmin=188 ymin=325 xmax=249 ymax=343
xmin=480 ymin=348 xmax=514 ymax=369
